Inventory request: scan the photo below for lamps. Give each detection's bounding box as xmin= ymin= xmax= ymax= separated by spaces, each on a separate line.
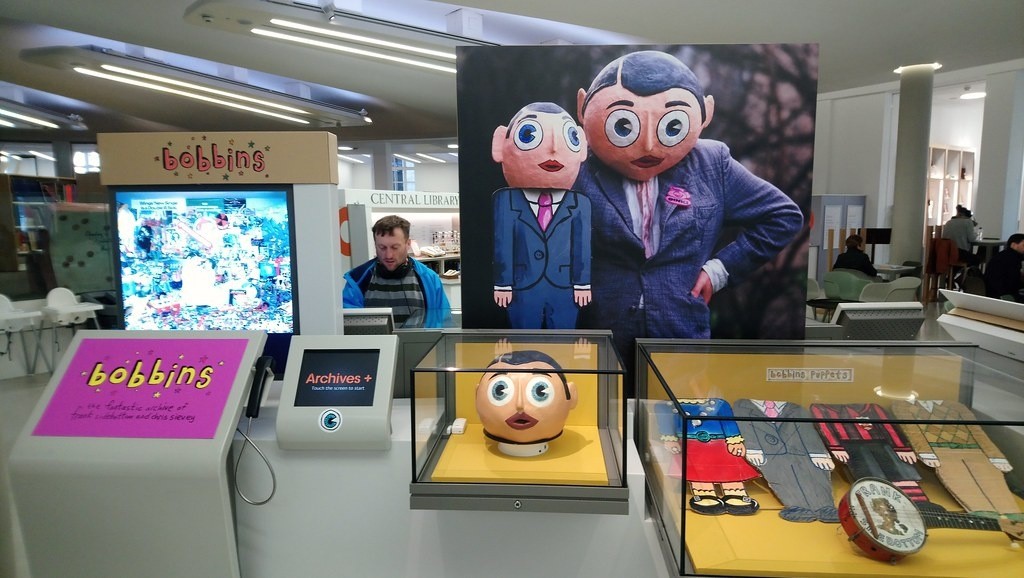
xmin=0 ymin=98 xmax=88 ymax=132
xmin=182 ymin=0 xmax=508 ymax=78
xmin=20 ymin=45 xmax=373 ymax=128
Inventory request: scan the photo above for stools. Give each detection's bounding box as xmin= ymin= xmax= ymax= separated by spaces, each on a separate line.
xmin=0 ymin=294 xmax=53 ymax=377
xmin=33 ymin=286 xmax=105 ymax=375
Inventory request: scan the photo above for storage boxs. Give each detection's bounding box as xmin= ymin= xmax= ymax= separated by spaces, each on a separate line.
xmin=410 ymin=330 xmax=630 ymax=513
xmin=634 ymin=338 xmax=1024 ymax=578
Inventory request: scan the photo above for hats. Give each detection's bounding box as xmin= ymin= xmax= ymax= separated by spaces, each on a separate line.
xmin=956 ymin=205 xmax=972 ymax=218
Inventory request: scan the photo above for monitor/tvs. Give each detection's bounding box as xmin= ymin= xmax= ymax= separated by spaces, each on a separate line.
xmin=110 ymin=184 xmax=300 ymax=336
xmin=292 ymin=349 xmax=380 ymax=407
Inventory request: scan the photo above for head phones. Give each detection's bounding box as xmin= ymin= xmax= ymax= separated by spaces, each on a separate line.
xmin=376 ymin=257 xmax=412 ymax=278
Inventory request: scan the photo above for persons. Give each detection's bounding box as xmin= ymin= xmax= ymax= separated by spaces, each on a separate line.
xmin=343 ymin=215 xmax=451 ymax=329
xmin=943 ymin=208 xmax=977 ymax=288
xmin=833 ymin=235 xmax=877 ymax=277
xmin=572 ymin=138 xmax=805 ymax=398
xmin=984 ymin=233 xmax=1024 ymax=303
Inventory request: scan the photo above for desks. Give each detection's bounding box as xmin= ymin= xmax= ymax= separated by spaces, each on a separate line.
xmin=973 ymin=239 xmax=1007 ymax=256
xmin=807 ymin=298 xmax=863 ymax=323
xmin=413 ymin=255 xmax=461 ymax=273
xmin=875 ymin=266 xmax=915 ymax=281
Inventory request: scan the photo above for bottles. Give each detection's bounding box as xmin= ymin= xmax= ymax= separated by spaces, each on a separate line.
xmin=977 ymin=227 xmax=983 ymax=241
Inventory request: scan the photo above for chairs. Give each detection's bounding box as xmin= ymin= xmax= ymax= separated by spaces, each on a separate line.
xmin=807 ymin=238 xmax=967 ymax=302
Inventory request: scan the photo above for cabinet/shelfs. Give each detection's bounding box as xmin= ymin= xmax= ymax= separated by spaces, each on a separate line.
xmin=923 ymin=147 xmax=975 ymax=244
